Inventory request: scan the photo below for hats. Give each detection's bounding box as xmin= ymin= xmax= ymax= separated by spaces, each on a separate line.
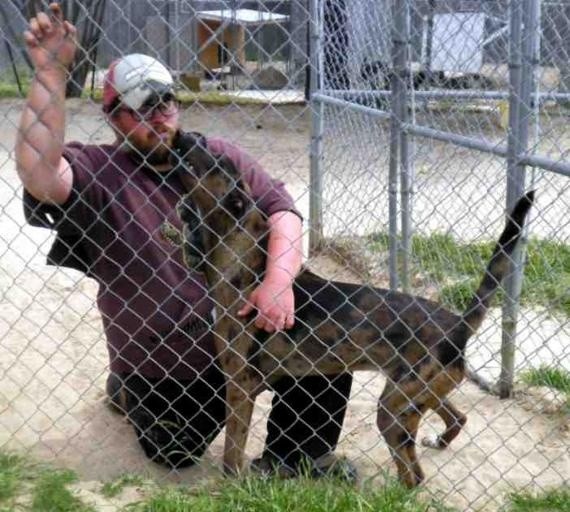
xmin=103 ymin=53 xmax=174 ymax=111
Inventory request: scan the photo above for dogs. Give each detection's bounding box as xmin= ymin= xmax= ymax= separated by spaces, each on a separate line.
xmin=168 ymin=132 xmax=535 ymax=493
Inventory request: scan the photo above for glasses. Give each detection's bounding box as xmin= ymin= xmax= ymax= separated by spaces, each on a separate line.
xmin=118 ymin=98 xmax=182 ymax=122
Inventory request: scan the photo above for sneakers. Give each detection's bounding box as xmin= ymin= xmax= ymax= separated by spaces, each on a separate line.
xmin=259 ymin=455 xmax=357 ymax=485
xmin=107 ymin=372 xmax=128 ymax=413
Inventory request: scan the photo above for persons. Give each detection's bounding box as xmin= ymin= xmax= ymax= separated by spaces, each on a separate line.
xmin=11 ymin=1 xmax=356 ymax=478
